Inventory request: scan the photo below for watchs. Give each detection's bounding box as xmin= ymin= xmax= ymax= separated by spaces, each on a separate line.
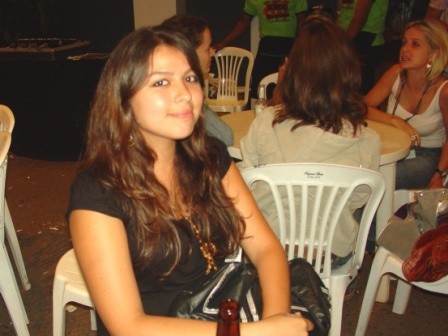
xmin=437 ymin=169 xmax=446 ymax=177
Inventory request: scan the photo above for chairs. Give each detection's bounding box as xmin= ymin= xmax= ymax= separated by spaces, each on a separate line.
xmin=0 ymin=104 xmax=31 ymax=336
xmin=353 ymin=245 xmax=448 ymax=336
xmin=52 ymin=247 xmax=97 ymax=336
xmin=238 ymin=162 xmax=385 ymax=336
xmin=205 ymin=47 xmax=254 ymax=115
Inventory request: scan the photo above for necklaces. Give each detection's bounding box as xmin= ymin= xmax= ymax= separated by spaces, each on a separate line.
xmin=392 ymin=79 xmax=430 ymax=122
xmin=179 ymin=209 xmax=218 ymax=273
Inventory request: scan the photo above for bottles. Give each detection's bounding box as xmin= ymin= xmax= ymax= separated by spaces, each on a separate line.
xmin=254 ymin=84 xmax=268 ymax=118
xmin=216 ymin=298 xmax=241 ymax=336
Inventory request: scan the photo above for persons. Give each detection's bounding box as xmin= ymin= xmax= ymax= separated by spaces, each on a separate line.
xmin=337 ymin=0 xmax=388 ymax=96
xmin=236 ymin=17 xmax=379 ymax=299
xmin=65 ymin=27 xmax=316 ymax=336
xmin=163 ymin=13 xmax=215 ymax=78
xmin=213 ymin=0 xmax=308 ymax=100
xmin=365 ymin=21 xmax=448 ymax=190
xmin=425 ymin=0 xmax=448 ymax=25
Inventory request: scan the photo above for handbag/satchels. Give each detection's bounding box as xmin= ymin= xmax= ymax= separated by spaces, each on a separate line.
xmin=176 ymin=257 xmax=333 ymax=336
xmin=376 ymin=188 xmax=448 ymax=261
xmin=402 ymin=223 xmax=448 ymax=282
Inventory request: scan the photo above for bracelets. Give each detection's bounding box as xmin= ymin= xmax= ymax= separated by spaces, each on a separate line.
xmin=216 ymin=298 xmax=240 ymax=336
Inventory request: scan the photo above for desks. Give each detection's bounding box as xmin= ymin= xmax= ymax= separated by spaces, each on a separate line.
xmin=221 ymin=109 xmax=414 ymax=302
